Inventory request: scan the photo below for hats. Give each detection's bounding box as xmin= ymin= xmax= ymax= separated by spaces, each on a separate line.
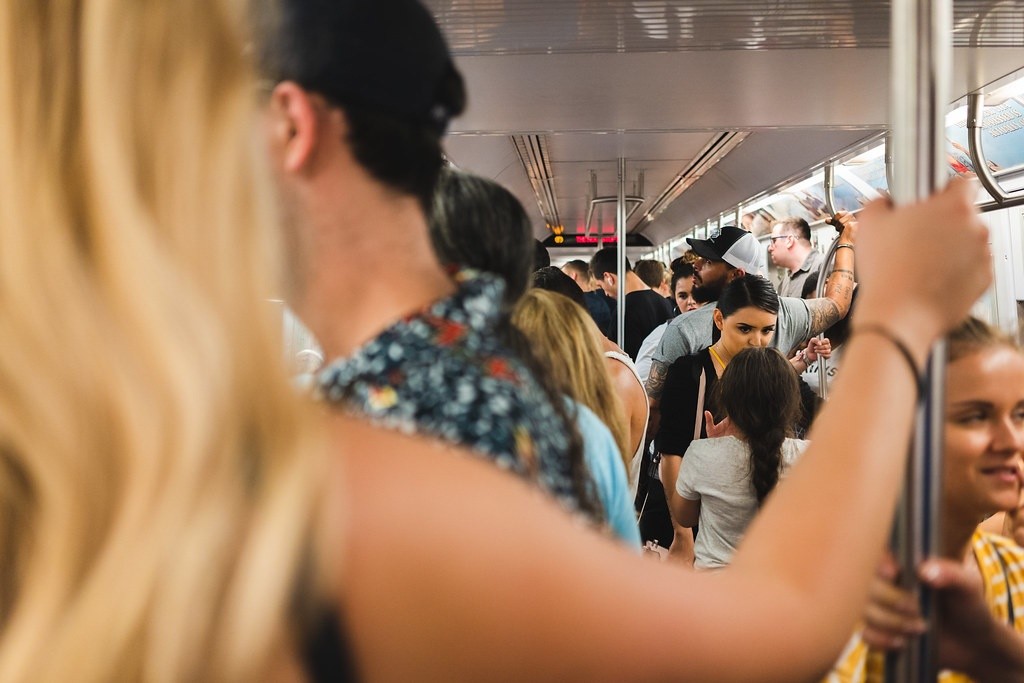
xmin=686 ymin=225 xmax=765 ymax=278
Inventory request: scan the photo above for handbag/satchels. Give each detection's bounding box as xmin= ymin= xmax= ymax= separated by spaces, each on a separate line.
xmin=641 ymin=540 xmax=669 ymax=571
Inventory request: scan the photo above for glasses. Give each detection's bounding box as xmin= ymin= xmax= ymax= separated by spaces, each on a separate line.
xmin=770 ymin=235 xmax=798 ymax=244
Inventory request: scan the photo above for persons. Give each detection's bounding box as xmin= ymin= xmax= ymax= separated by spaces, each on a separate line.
xmin=0 ymin=1 xmax=857 ymax=683
xmin=820 ymin=317 xmax=1023 ymax=683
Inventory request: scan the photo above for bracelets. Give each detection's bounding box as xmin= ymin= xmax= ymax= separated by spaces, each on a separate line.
xmin=836 ymin=244 xmax=855 ymax=251
xmin=849 ymin=322 xmax=924 ymax=405
